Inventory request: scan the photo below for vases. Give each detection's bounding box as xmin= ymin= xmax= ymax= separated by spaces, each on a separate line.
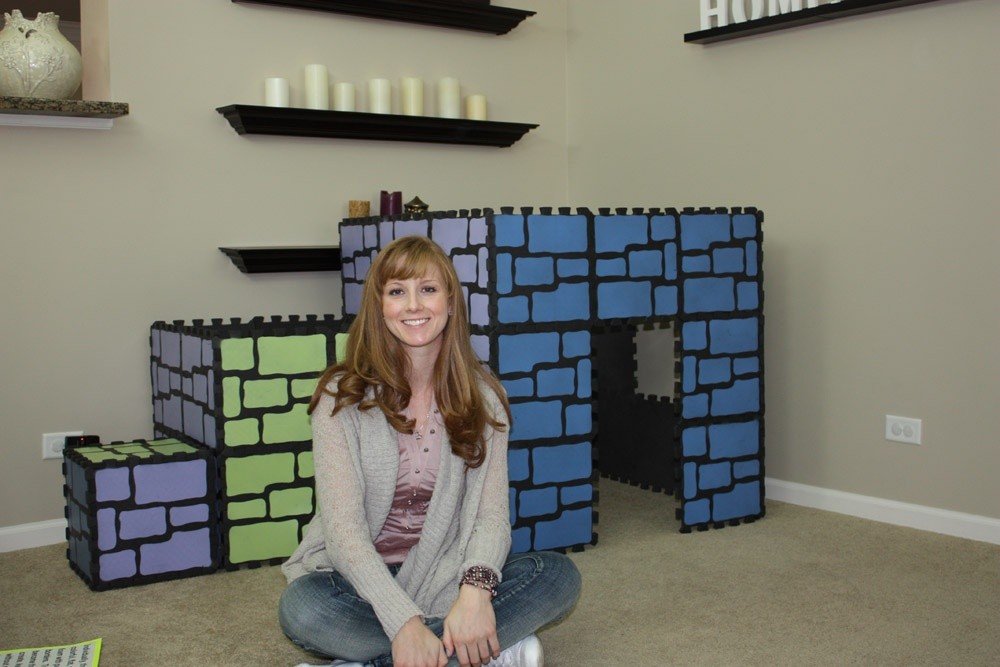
xmin=0 ymin=8 xmax=84 ymax=100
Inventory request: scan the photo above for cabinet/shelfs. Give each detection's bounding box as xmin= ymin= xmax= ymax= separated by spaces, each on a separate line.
xmin=215 ymin=1 xmax=541 ymax=275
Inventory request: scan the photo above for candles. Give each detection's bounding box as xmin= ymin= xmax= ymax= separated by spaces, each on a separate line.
xmin=304 ymin=64 xmax=328 ymax=110
xmin=265 ymin=77 xmax=289 ymax=107
xmin=367 ymin=79 xmax=392 ymax=114
xmin=333 ymin=83 xmax=356 ymax=112
xmin=465 ymin=94 xmax=486 ymax=121
xmin=400 ymin=78 xmax=424 ymax=116
xmin=436 ymin=77 xmax=460 ymax=119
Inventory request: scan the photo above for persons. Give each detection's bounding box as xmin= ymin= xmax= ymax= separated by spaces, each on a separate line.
xmin=278 ymin=234 xmax=581 ymax=667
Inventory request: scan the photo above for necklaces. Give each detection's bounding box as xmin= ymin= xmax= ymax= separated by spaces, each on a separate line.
xmin=416 ymin=409 xmax=431 ymax=432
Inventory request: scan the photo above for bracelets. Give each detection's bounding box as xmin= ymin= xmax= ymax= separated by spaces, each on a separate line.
xmin=457 ymin=565 xmax=499 ymax=598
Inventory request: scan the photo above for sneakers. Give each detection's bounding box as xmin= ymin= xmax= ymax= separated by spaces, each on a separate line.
xmin=473 ymin=632 xmax=544 ymax=667
xmin=297 ymin=658 xmax=372 ymax=667
